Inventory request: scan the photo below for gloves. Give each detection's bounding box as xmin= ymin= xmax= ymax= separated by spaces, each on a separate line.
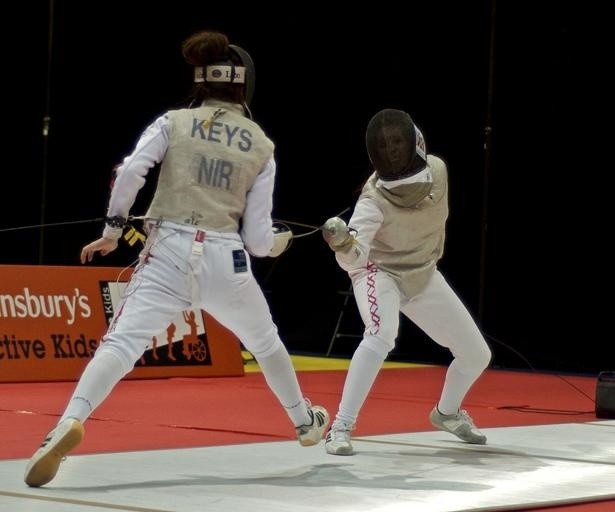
xmin=328 ymin=235 xmax=354 ymax=254
xmin=269 ymin=227 xmax=294 ymax=258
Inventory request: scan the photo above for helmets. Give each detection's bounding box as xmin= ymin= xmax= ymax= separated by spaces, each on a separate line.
xmin=365 ymin=109 xmax=433 ymax=209
xmin=183 ymin=32 xmax=257 ymax=122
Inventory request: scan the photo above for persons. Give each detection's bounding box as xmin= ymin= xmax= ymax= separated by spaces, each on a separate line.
xmin=24 ymin=31 xmax=330 ymax=487
xmin=325 ymin=108 xmax=493 ymax=456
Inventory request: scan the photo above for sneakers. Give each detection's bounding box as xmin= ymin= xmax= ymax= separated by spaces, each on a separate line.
xmin=295 ymin=397 xmax=330 ymax=447
xmin=324 ymin=428 xmax=355 ymax=455
xmin=24 ymin=416 xmax=85 ymax=488
xmin=429 ymin=403 xmax=486 ymax=444
